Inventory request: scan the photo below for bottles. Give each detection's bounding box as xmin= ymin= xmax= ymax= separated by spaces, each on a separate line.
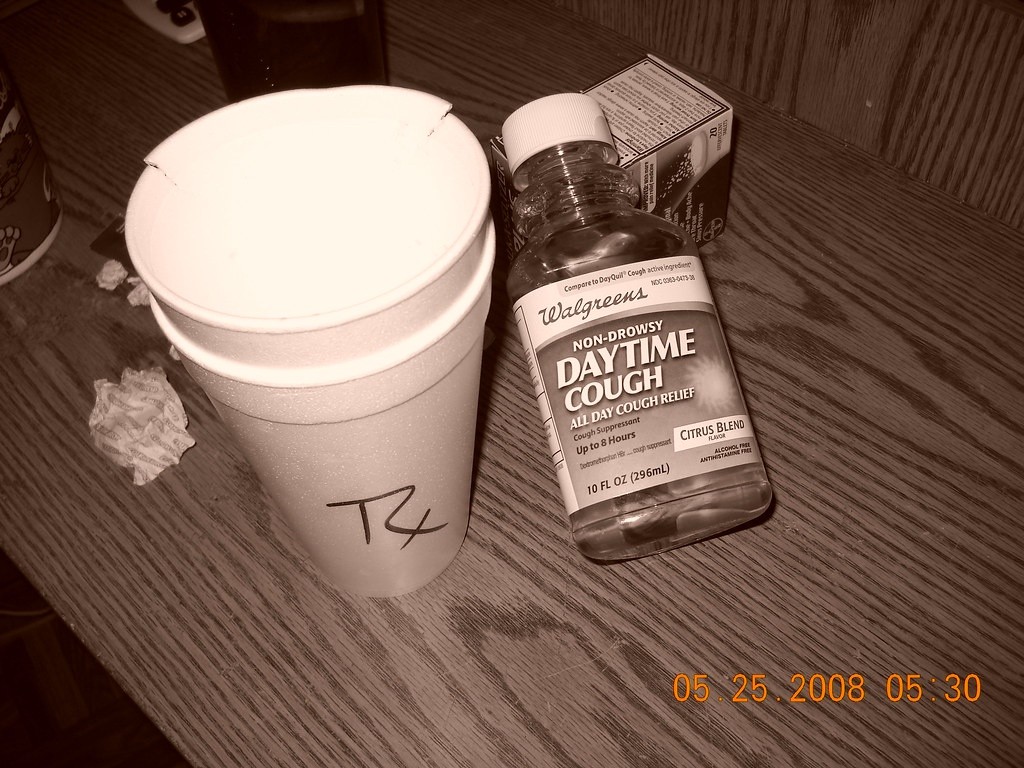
xmin=504 ymin=91 xmax=774 ymax=564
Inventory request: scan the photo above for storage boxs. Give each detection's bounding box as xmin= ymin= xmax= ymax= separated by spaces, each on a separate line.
xmin=485 ymin=50 xmax=736 ymax=284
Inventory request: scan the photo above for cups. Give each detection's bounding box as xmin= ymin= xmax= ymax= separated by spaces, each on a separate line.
xmin=126 ymin=83 xmax=491 ymax=375
xmin=194 ymin=0 xmax=389 ymax=112
xmin=148 ymin=221 xmax=497 ymax=597
xmin=0 ymin=69 xmax=63 ymax=287
